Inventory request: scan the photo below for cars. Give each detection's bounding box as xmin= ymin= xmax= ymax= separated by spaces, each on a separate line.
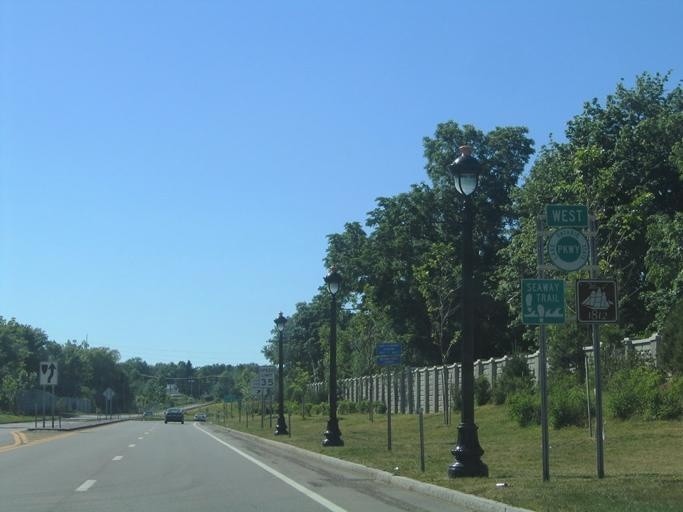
xmin=192 ymin=413 xmax=208 ymax=422
xmin=143 ymin=410 xmax=153 ymax=417
xmin=163 ymin=408 xmax=184 ymax=424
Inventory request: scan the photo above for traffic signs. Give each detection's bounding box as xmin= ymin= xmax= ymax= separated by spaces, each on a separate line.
xmin=545 ymin=205 xmax=587 ymax=227
xmin=258 ymin=366 xmax=275 ymax=388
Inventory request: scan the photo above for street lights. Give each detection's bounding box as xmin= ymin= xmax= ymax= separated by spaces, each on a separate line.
xmin=317 ymin=266 xmax=348 ymax=448
xmin=445 ymin=138 xmax=487 ymax=482
xmin=273 ymin=310 xmax=291 ymax=436
